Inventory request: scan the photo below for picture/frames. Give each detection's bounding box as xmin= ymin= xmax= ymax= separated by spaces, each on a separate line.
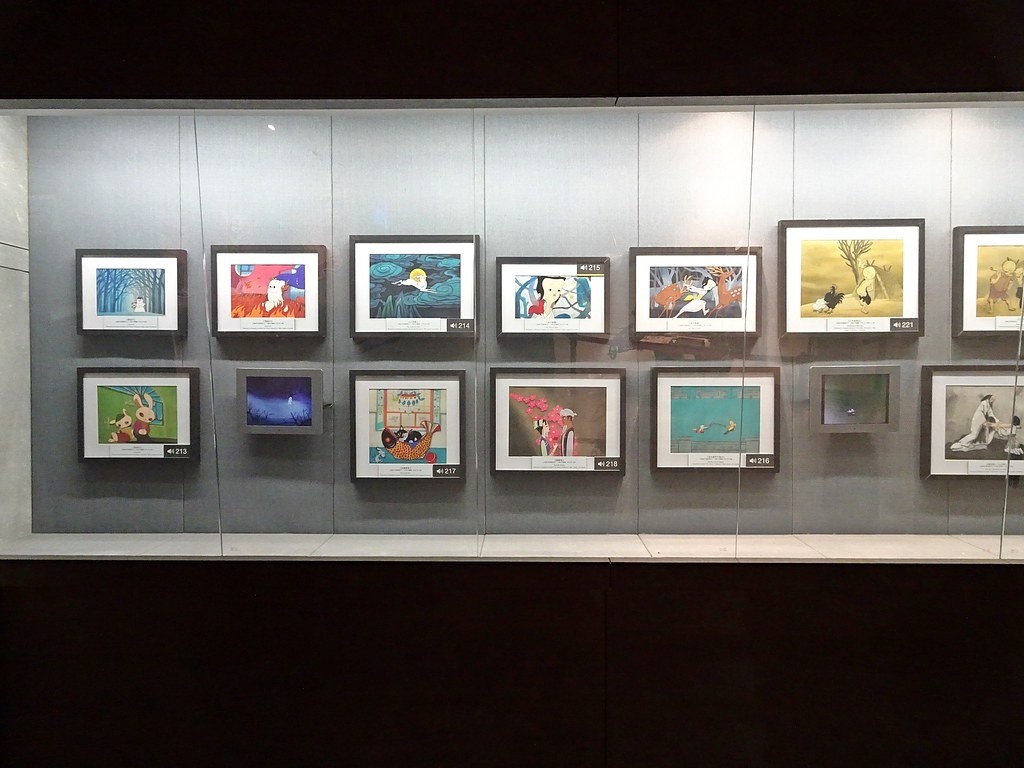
xmin=777 ymin=217 xmax=926 ymax=339
xmin=210 ymin=245 xmax=326 ymax=339
xmin=234 ymin=367 xmax=323 ymax=436
xmin=76 ymin=367 xmax=201 ymax=466
xmin=348 ymin=369 xmax=466 ymax=486
xmin=75 ymin=248 xmax=188 ymax=338
xmin=808 ymin=365 xmax=900 ymax=433
xmin=628 ymin=246 xmax=762 ymax=343
xmin=490 ymin=367 xmax=626 ymax=478
xmin=650 ymin=365 xmax=780 ymax=474
xmin=495 ymin=256 xmax=611 ymax=340
xmin=951 ymin=226 xmax=1024 ymax=341
xmin=921 ymin=364 xmax=1024 ymax=481
xmin=349 ymin=234 xmax=480 ymax=339
